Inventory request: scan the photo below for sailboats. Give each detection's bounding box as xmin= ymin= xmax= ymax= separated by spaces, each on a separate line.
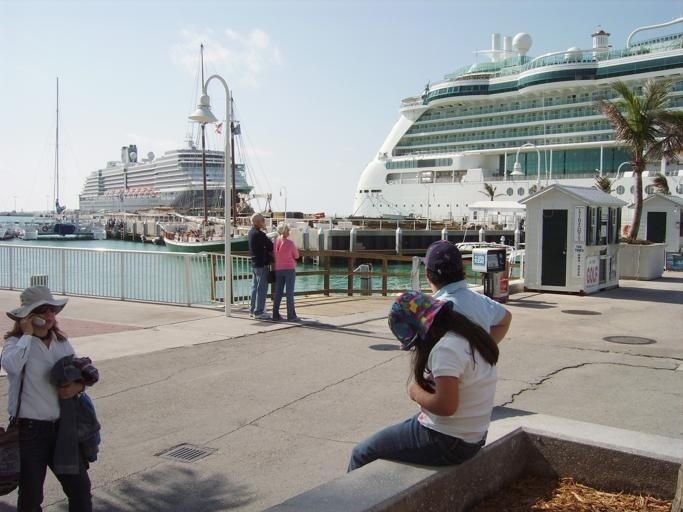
xmin=18 ymin=73 xmax=94 ymax=241
xmin=160 ymin=39 xmax=277 ymax=258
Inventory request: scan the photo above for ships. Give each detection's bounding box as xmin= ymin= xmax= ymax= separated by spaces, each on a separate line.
xmin=348 ymin=14 xmax=682 ymax=231
xmin=78 ymin=142 xmax=256 ymax=217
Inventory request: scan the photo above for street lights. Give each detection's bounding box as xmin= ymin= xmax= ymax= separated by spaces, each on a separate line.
xmin=279 ymin=185 xmax=289 ymax=222
xmin=507 ymin=141 xmax=541 ymax=192
xmin=186 ymin=72 xmax=233 ymax=317
xmin=419 ymin=170 xmax=434 ymax=231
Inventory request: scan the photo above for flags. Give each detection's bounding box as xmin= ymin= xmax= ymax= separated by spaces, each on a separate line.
xmin=231 ymin=124 xmax=243 ymax=135
xmin=214 ymin=122 xmax=224 ymax=134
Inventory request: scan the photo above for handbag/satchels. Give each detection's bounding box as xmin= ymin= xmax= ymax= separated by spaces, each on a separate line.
xmin=268 ymin=270 xmax=276 ymax=283
xmin=0 ymin=418 xmax=21 ymax=495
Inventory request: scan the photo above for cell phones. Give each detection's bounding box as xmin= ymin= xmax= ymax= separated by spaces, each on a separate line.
xmin=31 ymin=314 xmax=47 ymax=327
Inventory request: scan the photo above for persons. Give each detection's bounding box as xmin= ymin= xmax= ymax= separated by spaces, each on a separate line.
xmin=1 ymin=284 xmax=96 ymax=512
xmin=246 ymin=212 xmax=274 ymax=322
xmin=421 ymin=240 xmax=514 ymax=346
xmin=271 ymin=221 xmax=301 ymax=323
xmin=343 ymin=289 xmax=498 ymax=473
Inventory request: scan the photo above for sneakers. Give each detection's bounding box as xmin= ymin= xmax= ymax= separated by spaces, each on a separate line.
xmin=249 ymin=308 xmax=302 ymax=321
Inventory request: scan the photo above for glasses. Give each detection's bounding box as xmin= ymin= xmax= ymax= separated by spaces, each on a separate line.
xmin=31 ymin=304 xmax=57 ymax=314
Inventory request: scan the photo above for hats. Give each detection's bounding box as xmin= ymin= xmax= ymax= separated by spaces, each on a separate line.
xmin=425 ymin=239 xmax=463 ymax=275
xmin=5 ymin=285 xmax=69 ymax=322
xmin=388 ymin=291 xmax=453 ymax=351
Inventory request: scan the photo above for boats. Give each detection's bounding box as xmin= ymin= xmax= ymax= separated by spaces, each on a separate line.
xmin=0 ymin=210 xmax=57 ymax=242
xmin=452 ymin=239 xmax=528 ymax=265
